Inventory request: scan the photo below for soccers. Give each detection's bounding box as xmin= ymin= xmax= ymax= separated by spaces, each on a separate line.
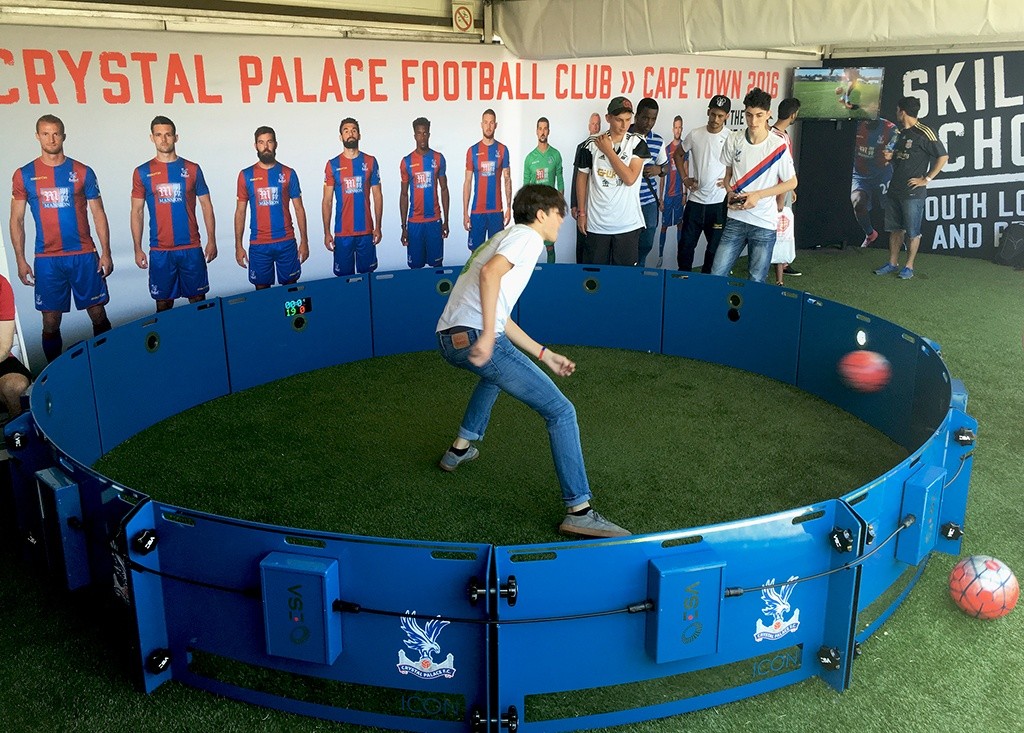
xmin=945 ymin=552 xmax=1020 ymax=624
xmin=836 ymin=349 xmax=891 ymax=394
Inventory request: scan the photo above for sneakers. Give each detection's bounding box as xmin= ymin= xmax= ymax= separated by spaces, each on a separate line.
xmin=897 ymin=266 xmax=914 ymax=279
xmin=861 ymin=230 xmax=878 ymax=247
xmin=783 ymin=266 xmax=802 ymax=276
xmin=439 ymin=443 xmax=480 ymax=470
xmin=561 ymin=508 xmax=632 ymax=537
xmin=873 ymin=262 xmax=898 ymax=275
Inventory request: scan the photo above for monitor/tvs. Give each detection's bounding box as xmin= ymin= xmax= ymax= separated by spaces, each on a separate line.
xmin=790 ymin=66 xmax=886 ymax=122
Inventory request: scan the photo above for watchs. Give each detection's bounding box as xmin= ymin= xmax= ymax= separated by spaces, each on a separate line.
xmin=658 ymin=165 xmax=666 ymax=176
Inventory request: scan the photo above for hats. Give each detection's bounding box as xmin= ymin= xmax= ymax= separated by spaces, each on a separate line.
xmin=608 ymin=97 xmax=635 ymax=114
xmin=708 ymin=94 xmax=731 ymax=114
xmin=770 ymin=116 xmax=773 ymax=119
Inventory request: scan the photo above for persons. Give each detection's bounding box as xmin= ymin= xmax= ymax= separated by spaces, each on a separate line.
xmin=657 ymin=115 xmax=690 ymax=267
xmin=234 ymin=126 xmax=310 ymax=295
xmin=399 ymin=116 xmax=450 ymax=274
xmin=873 ymin=95 xmax=950 ymax=279
xmin=130 ymin=115 xmax=217 ymax=312
xmin=0 ymin=274 xmax=33 ymax=418
xmin=463 ymin=108 xmax=512 ymax=255
xmin=437 ymin=185 xmax=632 ymax=537
xmin=9 ymin=114 xmax=115 ymax=367
xmin=524 ymin=117 xmax=565 ymax=264
xmin=628 ymin=96 xmax=671 ymax=267
xmin=839 ymin=67 xmax=859 ymax=109
xmin=673 ymin=95 xmax=732 ymax=274
xmin=710 ymin=87 xmax=797 ymax=283
xmin=769 ymin=97 xmax=803 ymax=276
xmin=850 ymin=96 xmax=900 ymax=247
xmin=570 ymin=113 xmax=601 ymax=263
xmin=575 ymin=96 xmax=651 ymax=266
xmin=322 ymin=118 xmax=383 ymax=282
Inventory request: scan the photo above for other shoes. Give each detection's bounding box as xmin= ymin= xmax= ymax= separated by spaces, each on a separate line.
xmin=656 ymin=257 xmax=663 ymax=268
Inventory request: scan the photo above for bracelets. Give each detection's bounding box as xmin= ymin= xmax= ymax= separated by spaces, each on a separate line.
xmin=925 ymin=175 xmax=932 ymax=182
xmin=538 ymin=346 xmax=547 ymax=360
xmin=683 ymin=192 xmax=687 ymax=196
xmin=727 ymin=191 xmax=733 ymax=195
xmin=577 ymin=211 xmax=587 ymax=217
xmin=681 ymin=175 xmax=688 ymax=183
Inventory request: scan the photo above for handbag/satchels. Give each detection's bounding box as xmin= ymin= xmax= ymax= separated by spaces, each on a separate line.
xmin=771 ymin=206 xmax=796 ymax=264
